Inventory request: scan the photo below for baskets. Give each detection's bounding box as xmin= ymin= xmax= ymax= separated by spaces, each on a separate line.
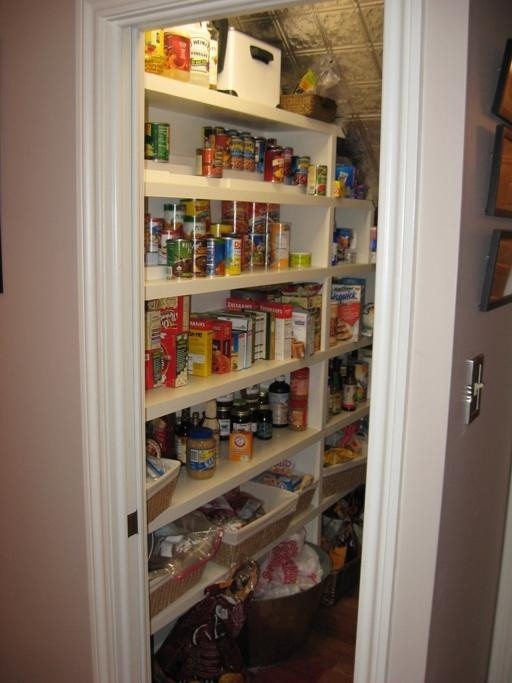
xmin=192 ymin=480 xmax=299 ymax=567
xmin=149 ymin=537 xmax=207 ymax=617
xmin=251 ymin=474 xmax=318 ymax=516
xmin=322 ymin=456 xmax=367 ymax=497
xmin=281 ymin=94 xmax=337 ymax=122
xmin=147 ymin=456 xmax=181 ymax=524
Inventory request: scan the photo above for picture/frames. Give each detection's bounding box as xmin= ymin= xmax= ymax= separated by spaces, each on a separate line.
xmin=493 ymin=38 xmax=512 ymax=125
xmin=485 ymin=122 xmax=512 ymax=218
xmin=480 ymin=227 xmax=512 ymax=311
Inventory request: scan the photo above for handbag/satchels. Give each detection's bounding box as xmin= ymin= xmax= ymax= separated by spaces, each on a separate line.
xmin=155 ymin=558 xmax=259 ymax=680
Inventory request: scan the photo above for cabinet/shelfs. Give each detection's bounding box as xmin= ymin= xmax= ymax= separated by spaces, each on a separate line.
xmin=145 ymin=71 xmax=379 ymax=648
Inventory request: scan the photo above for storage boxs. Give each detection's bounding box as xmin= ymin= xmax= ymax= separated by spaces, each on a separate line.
xmin=214 ymin=26 xmax=282 ymax=111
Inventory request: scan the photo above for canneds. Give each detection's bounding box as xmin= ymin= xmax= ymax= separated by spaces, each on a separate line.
xmin=216 ymin=382 xmax=260 ymax=441
xmin=186 ymin=428 xmax=215 ymax=479
xmin=145 ymin=121 xmax=312 ymax=280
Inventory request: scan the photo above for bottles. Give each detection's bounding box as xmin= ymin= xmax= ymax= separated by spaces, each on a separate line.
xmin=187 ymin=18 xmax=219 ymax=89
xmin=342 ymin=364 xmax=358 ymax=410
xmin=328 ymin=359 xmax=342 ymax=414
xmin=175 ymin=399 xmax=220 ymax=464
xmin=268 ymin=373 xmax=290 ymax=426
